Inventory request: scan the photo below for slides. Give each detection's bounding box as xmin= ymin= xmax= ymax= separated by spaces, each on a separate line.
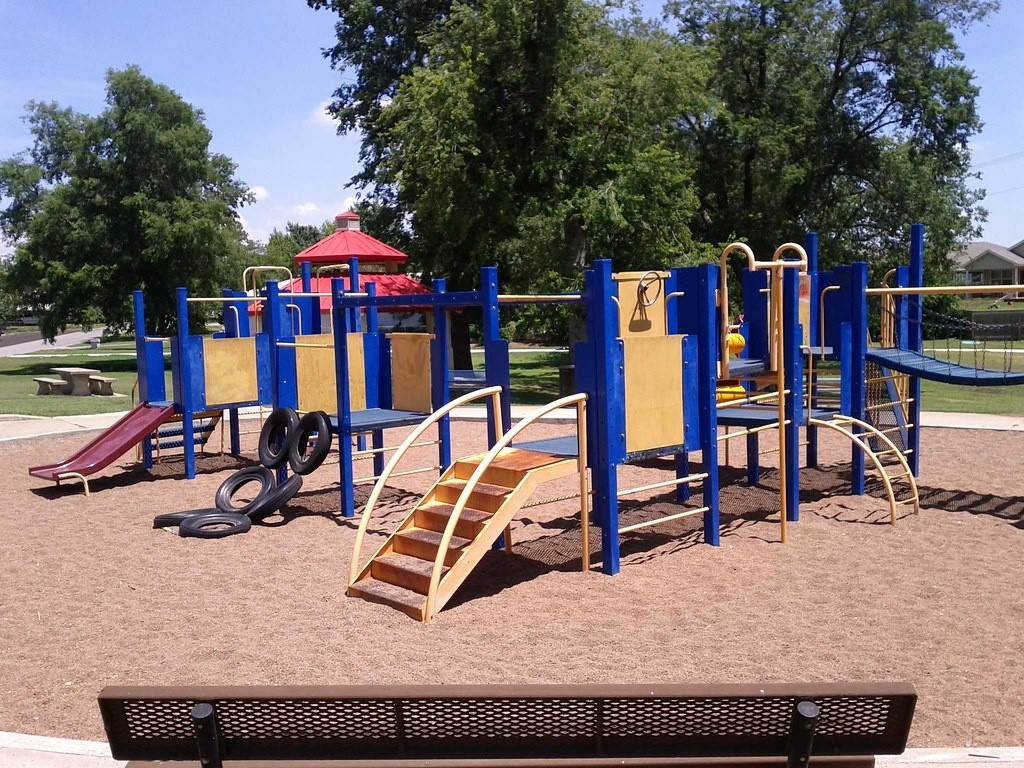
xmin=28 ymin=399 xmax=174 ymax=482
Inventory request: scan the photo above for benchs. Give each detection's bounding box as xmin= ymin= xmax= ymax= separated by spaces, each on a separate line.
xmin=96 ymin=684 xmax=918 ymax=768
xmin=32 ymin=377 xmax=68 ymax=395
xmin=90 ymin=338 xmax=100 ymax=349
xmin=89 ymin=376 xmax=117 ymax=396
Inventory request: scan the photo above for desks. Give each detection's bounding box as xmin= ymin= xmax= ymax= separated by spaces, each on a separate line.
xmin=50 ymin=368 xmax=100 ymax=395
